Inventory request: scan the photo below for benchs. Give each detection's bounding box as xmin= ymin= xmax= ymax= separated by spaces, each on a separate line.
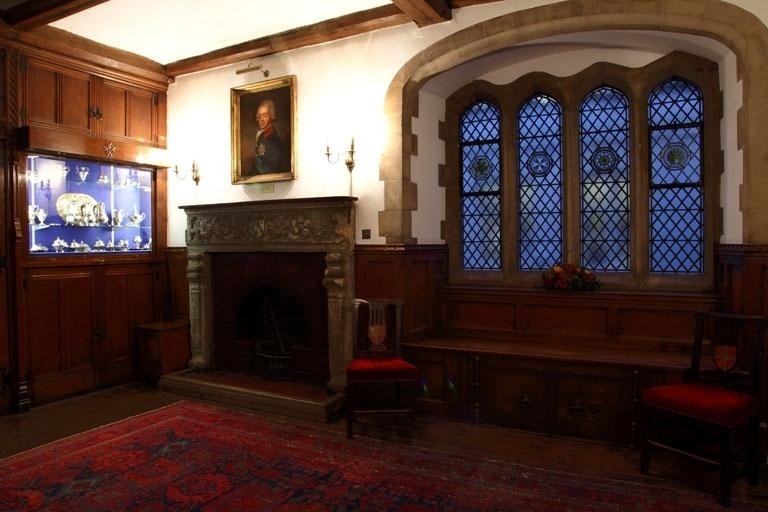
xmin=402 ymin=334 xmax=750 ymax=451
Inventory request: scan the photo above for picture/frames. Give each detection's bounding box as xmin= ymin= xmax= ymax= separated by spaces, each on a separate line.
xmin=229 ymin=74 xmax=298 ymax=186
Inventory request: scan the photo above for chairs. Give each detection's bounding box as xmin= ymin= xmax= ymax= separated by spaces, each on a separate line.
xmin=342 ymin=296 xmax=421 ymax=442
xmin=637 ymin=312 xmax=766 ymax=505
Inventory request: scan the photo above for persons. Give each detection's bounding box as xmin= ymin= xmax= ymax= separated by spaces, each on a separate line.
xmin=248 ymin=99 xmax=283 ymax=174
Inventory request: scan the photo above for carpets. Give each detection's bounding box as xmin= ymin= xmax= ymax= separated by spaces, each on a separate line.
xmin=0 ymin=396 xmax=766 ymax=512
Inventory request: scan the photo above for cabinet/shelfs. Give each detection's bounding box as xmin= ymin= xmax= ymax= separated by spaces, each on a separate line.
xmin=21 ymin=53 xmax=159 ymax=146
xmin=28 ymin=155 xmax=155 ymax=255
xmin=29 ymin=268 xmax=160 ymax=401
